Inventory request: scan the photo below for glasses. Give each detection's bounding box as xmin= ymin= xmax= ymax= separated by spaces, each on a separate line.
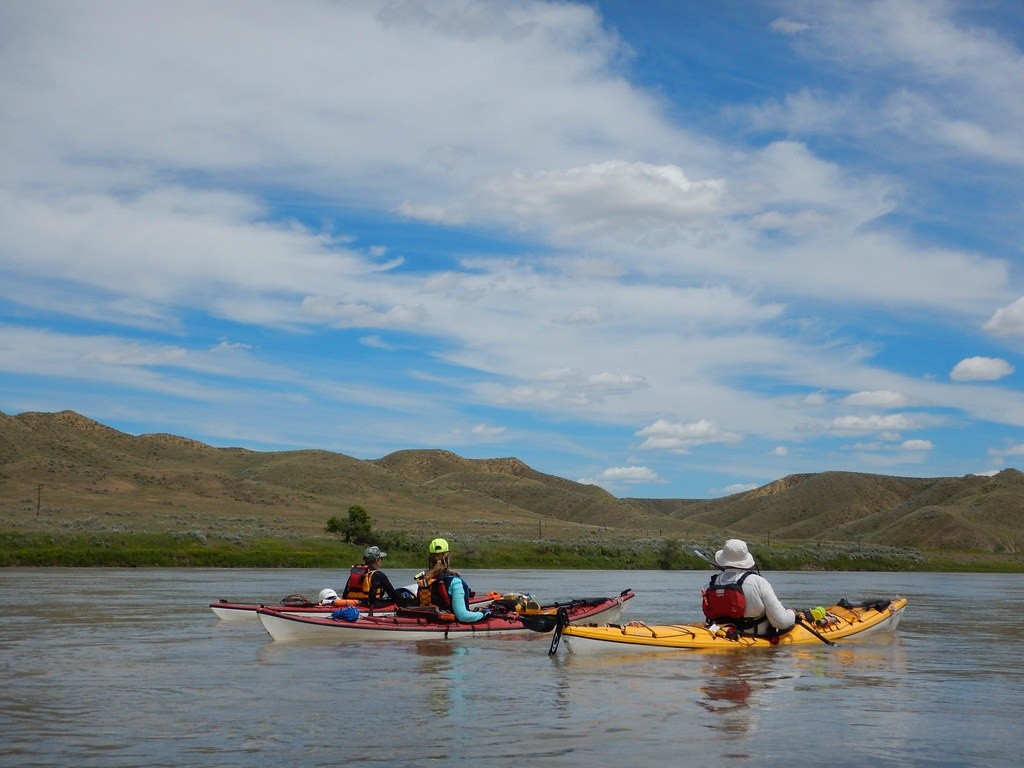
xmin=378 ymin=557 xmax=383 ymax=561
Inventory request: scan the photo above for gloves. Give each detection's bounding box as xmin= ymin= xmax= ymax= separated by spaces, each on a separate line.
xmin=481 ymin=609 xmax=492 ymax=616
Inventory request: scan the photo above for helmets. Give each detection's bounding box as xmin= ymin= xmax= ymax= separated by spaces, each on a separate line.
xmin=319 ymin=589 xmax=338 ymax=605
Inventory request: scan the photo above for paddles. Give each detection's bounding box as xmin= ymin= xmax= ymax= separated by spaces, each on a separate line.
xmin=391 ymin=587 xmax=558 ymax=633
xmin=694 ymin=549 xmax=838 ymax=646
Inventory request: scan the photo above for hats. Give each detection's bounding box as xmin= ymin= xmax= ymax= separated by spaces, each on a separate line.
xmin=429 ymin=538 xmax=449 ymax=553
xmin=363 ymin=546 xmax=387 ymax=561
xmin=714 ymin=539 xmax=755 ymax=569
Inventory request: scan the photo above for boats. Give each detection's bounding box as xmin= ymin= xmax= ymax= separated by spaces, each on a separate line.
xmin=210 ymin=594 xmax=504 ymax=620
xmin=257 ymin=588 xmax=635 ymax=644
xmin=548 ymin=595 xmax=908 ymax=655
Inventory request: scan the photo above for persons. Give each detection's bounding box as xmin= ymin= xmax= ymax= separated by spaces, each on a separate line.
xmin=415 ymin=537 xmax=492 ymax=623
xmin=341 ymin=546 xmax=418 ymax=609
xmin=702 ymin=538 xmax=802 ymax=637
